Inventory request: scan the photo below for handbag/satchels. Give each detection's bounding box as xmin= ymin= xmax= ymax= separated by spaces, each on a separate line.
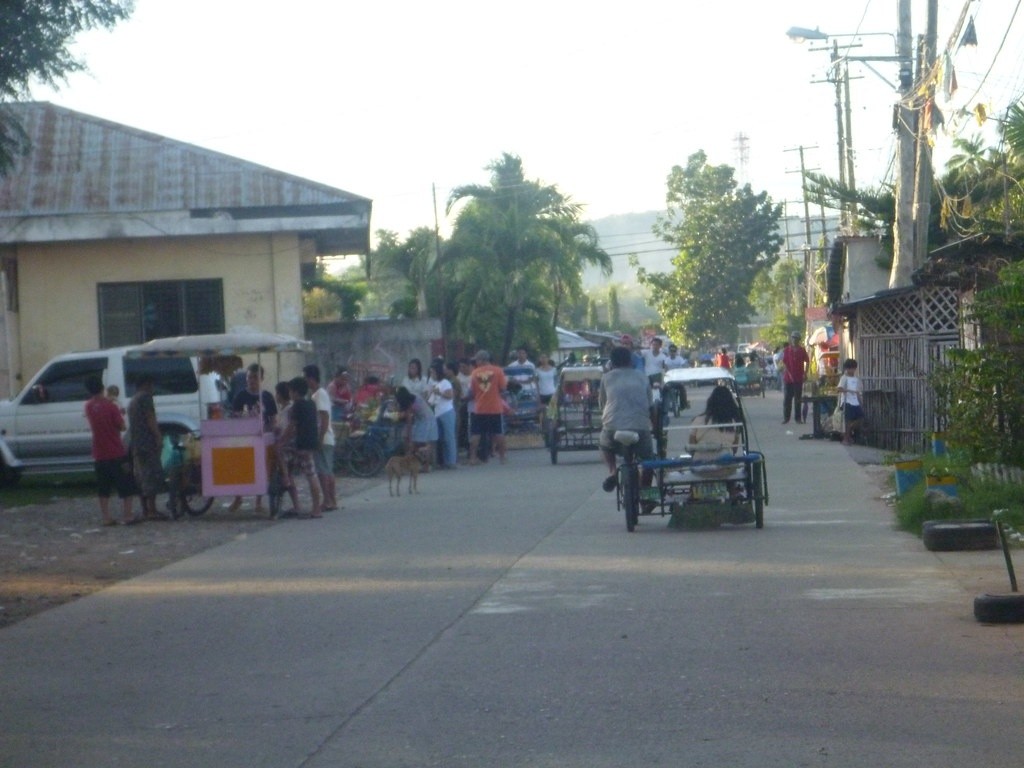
xmin=832 ymin=390 xmax=845 ymax=433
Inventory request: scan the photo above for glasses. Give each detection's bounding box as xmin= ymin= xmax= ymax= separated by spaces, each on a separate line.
xmin=791 ymin=336 xmax=798 ymax=339
xmin=670 ymin=350 xmax=676 ymax=353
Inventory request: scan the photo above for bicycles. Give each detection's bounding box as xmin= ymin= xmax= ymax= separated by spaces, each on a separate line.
xmin=326 ymin=412 xmax=384 ymax=475
xmin=648 ymin=377 xmax=664 ymax=429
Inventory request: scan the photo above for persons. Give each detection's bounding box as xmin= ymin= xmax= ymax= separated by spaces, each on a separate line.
xmin=397 ymin=386 xmax=439 ymax=472
xmin=560 ymin=335 xmax=688 ymax=407
xmin=508 ymin=348 xmax=537 ymax=390
xmin=227 ymin=364 xmax=277 ymax=515
xmin=401 ymin=357 xmax=478 ymax=470
xmin=536 ymin=353 xmax=559 ymax=405
xmin=837 ymin=359 xmax=864 ymax=445
xmin=688 ymin=387 xmax=741 ymax=504
xmin=129 ymin=374 xmax=169 ymax=520
xmin=85 ymin=376 xmax=137 ymax=525
xmin=274 ymin=364 xmax=338 ymax=518
xmin=701 ymin=346 xmax=778 ymax=392
xmin=328 ymin=367 xmax=384 ymax=407
xmin=774 ymin=330 xmax=809 ymax=425
xmin=471 ymin=350 xmax=507 ymax=463
xmin=599 ymin=346 xmax=659 ymax=514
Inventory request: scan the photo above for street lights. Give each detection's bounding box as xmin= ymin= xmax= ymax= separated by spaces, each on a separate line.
xmin=782 ymin=28 xmax=932 ymax=277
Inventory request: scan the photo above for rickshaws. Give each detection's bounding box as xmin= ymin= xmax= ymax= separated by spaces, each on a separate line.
xmin=478 ymin=367 xmax=550 ymax=452
xmin=547 ymin=363 xmax=606 ymax=464
xmin=719 ymin=353 xmax=766 ymax=398
xmin=611 ymin=365 xmax=769 ymax=534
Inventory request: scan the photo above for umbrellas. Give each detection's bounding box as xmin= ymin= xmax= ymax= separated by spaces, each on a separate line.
xmin=802 ymin=384 xmax=809 ymax=424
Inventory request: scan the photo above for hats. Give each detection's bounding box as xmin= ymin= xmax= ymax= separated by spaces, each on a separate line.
xmin=471 ymin=350 xmax=489 ymax=361
xmin=619 ymin=334 xmax=633 ymax=343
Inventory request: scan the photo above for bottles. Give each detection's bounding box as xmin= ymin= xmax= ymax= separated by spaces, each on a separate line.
xmin=241 ymin=404 xmax=250 ymax=418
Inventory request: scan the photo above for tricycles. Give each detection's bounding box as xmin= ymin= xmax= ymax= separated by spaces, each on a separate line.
xmin=143 ymin=336 xmax=312 ymax=520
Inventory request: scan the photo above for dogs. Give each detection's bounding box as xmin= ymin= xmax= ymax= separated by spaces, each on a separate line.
xmin=384 ymin=443 xmax=432 ymax=496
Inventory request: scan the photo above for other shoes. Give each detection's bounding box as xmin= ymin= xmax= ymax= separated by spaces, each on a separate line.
xmin=103 ymin=512 xmax=168 ymax=527
xmin=602 ymin=475 xmax=617 ymax=492
xmin=643 ymin=504 xmax=655 ymax=513
xmin=284 ymin=504 xmax=335 ymax=519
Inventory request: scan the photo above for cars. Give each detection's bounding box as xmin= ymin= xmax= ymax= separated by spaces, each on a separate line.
xmin=765 ymin=356 xmax=780 ymax=380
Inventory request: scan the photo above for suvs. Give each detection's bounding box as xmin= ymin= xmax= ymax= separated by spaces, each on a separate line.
xmin=1 ymin=343 xmax=230 ymax=488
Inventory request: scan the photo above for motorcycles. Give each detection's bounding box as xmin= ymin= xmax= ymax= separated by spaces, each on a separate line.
xmin=667 ymin=381 xmax=686 ymax=419
